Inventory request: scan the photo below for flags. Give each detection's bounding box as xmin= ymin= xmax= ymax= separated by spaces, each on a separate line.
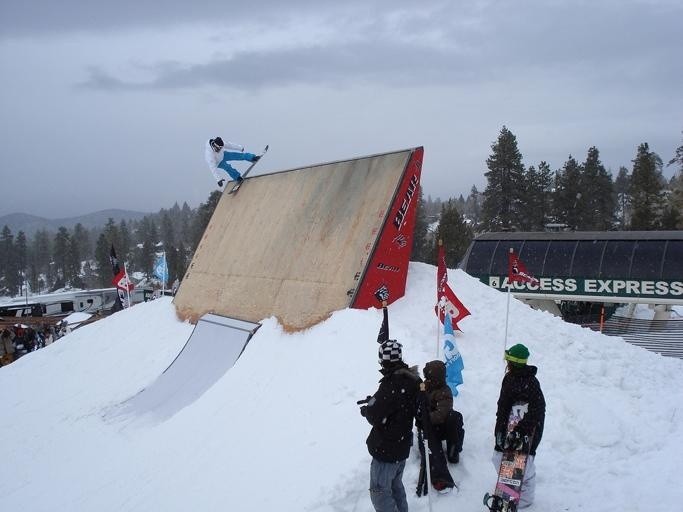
xmin=441 ymin=308 xmax=463 ymax=396
xmin=432 ymin=246 xmax=472 ymax=334
xmin=109 ymin=243 xmax=120 ymax=277
xmin=507 ymin=252 xmax=539 ymax=285
xmin=111 ymin=264 xmax=134 ymax=297
xmin=375 ymin=305 xmax=391 ymax=343
xmin=151 ymin=255 xmax=169 ymax=283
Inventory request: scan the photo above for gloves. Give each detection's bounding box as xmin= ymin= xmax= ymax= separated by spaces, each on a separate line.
xmin=217 ymin=179 xmax=225 ymax=186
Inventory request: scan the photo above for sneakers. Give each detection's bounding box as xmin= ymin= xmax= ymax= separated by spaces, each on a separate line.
xmin=236 ymin=176 xmax=244 ymax=182
xmin=254 ymin=156 xmax=260 ymax=161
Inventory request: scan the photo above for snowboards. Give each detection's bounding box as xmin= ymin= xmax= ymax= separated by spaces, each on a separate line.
xmin=227 ymin=145 xmax=268 ymax=195
xmin=483 ymin=421 xmax=537 ymax=512
xmin=415 ymin=391 xmax=458 ymax=494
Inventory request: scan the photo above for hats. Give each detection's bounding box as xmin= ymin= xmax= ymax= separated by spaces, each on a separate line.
xmin=378 ymin=340 xmax=402 ymax=365
xmin=214 ymin=137 xmax=223 ymax=147
xmin=504 ymin=343 xmax=529 ymax=364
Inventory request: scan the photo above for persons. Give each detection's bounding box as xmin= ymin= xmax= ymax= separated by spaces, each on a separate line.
xmin=490 ymin=341 xmax=546 ymax=509
xmin=411 ymin=358 xmax=465 ymax=453
xmin=0 ymin=320 xmax=71 ymax=370
xmin=358 ymin=338 xmax=422 ymax=512
xmin=203 ymin=134 xmax=261 ymax=187
